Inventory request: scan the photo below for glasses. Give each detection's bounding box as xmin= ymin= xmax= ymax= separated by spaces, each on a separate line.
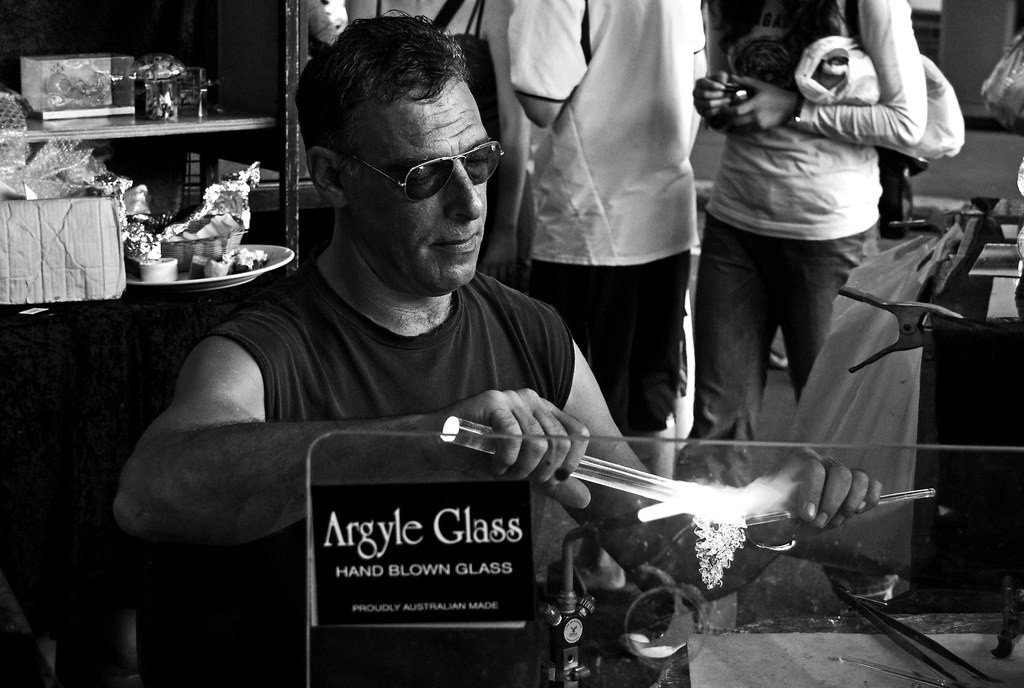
xmin=347 ymin=140 xmax=504 ymax=202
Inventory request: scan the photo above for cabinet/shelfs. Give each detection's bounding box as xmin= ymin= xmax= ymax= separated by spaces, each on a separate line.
xmin=0 ymin=1 xmax=336 ymax=277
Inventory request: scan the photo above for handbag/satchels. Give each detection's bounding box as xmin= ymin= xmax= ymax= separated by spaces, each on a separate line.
xmin=844 ymin=0 xmax=926 ymax=241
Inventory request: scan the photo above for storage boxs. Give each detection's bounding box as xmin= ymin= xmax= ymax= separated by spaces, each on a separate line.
xmin=0 ymin=194 xmax=125 ymax=306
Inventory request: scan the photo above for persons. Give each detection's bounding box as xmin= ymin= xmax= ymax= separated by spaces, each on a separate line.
xmin=507 ymin=0 xmax=707 ymax=588
xmin=674 ymin=0 xmax=929 ymax=487
xmin=115 ymin=13 xmax=882 ymax=580
